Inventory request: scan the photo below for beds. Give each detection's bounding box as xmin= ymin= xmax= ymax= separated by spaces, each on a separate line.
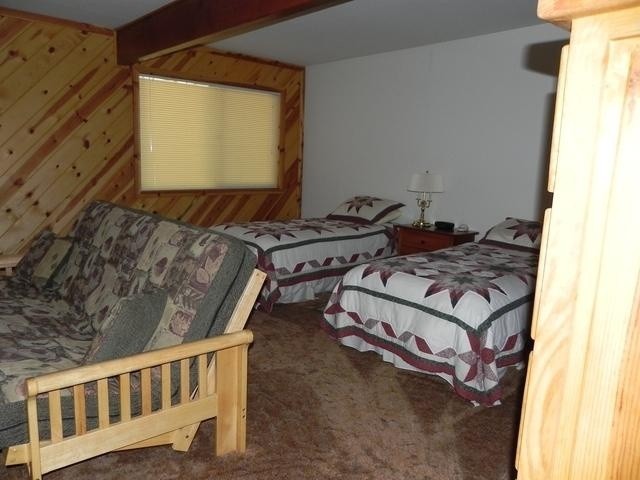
xmin=206 ymin=218 xmax=401 ymax=314
xmin=317 ymin=240 xmax=540 ymax=407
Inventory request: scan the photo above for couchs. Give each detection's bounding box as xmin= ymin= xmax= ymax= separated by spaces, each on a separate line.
xmin=0 ymin=200 xmax=268 ymax=480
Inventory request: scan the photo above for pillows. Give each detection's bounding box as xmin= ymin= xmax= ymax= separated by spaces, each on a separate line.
xmin=12 ymin=231 xmax=90 ymax=299
xmin=325 ymin=196 xmax=406 ymax=225
xmin=479 ymin=216 xmax=544 ymax=254
xmin=375 ymin=209 xmax=402 ymax=225
xmin=80 ymin=292 xmax=168 ymax=367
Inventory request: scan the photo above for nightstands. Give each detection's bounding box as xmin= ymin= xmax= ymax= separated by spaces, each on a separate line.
xmin=393 ymin=224 xmax=480 ymax=256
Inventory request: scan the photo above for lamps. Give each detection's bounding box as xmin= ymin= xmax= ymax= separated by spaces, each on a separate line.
xmin=406 ymin=170 xmax=444 ymax=227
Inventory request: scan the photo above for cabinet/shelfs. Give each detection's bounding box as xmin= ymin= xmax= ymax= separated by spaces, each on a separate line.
xmin=514 ymin=0 xmax=639 ymax=480
